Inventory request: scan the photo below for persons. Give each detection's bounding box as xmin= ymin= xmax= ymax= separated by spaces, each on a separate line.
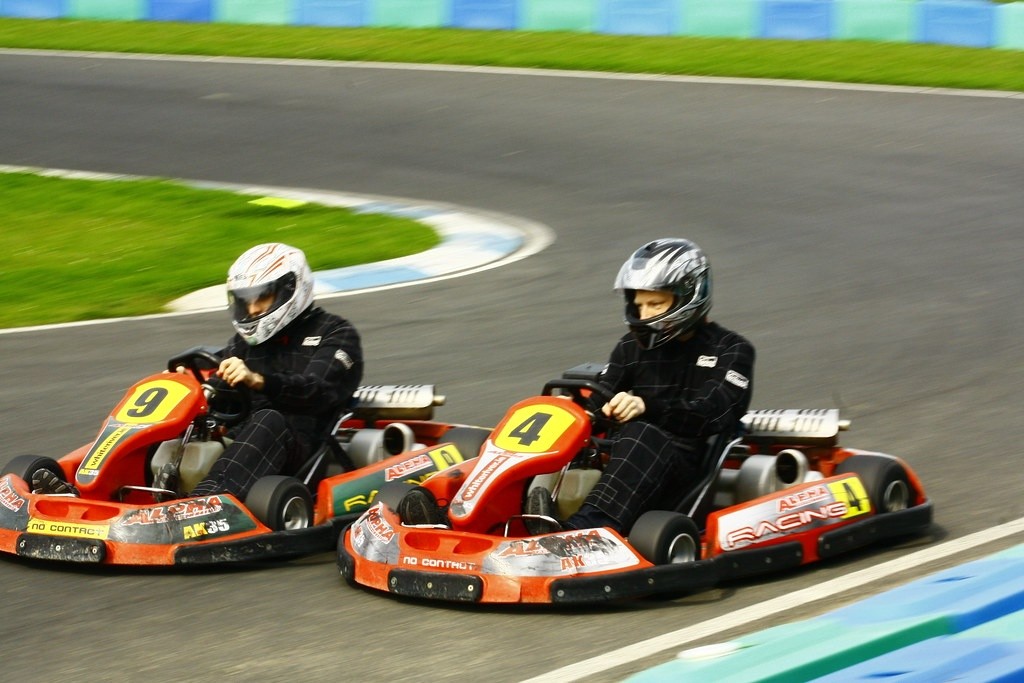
xmin=396 ymin=237 xmax=756 ymax=532
xmin=32 ymin=242 xmax=363 ymax=504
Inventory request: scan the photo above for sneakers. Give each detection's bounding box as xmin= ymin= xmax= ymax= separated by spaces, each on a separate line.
xmin=522 ymin=484 xmax=561 ymax=534
xmin=30 ymin=468 xmax=76 ymax=494
xmin=150 ymin=462 xmax=186 ymax=503
xmin=395 ymin=485 xmax=450 ymax=527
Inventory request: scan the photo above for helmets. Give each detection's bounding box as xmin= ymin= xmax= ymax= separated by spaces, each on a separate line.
xmin=225 ymin=242 xmax=314 ymax=347
xmin=611 ymin=235 xmax=711 ymax=349
